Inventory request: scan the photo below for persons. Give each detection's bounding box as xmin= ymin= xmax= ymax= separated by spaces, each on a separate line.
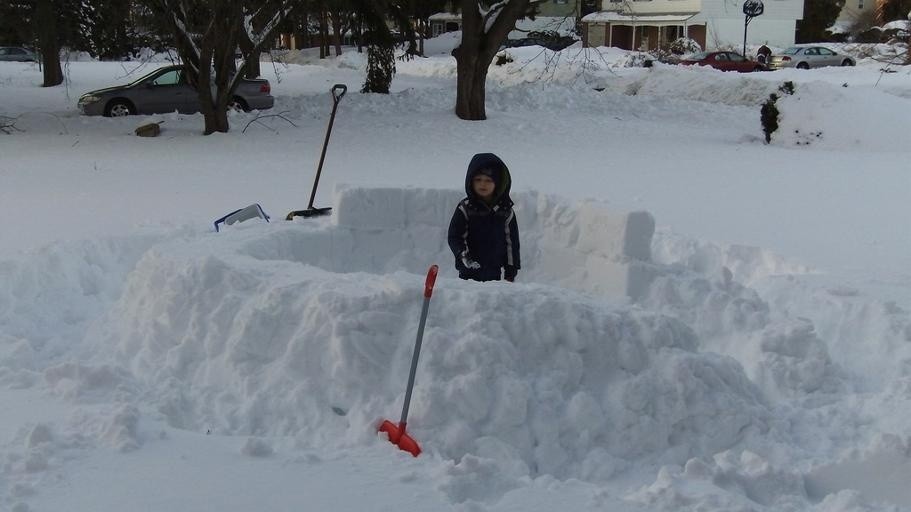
xmin=445 ymin=152 xmax=523 ymax=282
xmin=757 ymin=44 xmax=772 ymax=63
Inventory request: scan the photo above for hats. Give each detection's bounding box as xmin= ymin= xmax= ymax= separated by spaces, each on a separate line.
xmin=476 ymin=166 xmax=498 ymax=181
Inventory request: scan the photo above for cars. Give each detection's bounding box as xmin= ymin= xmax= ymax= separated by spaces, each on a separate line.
xmin=676 ymin=51 xmax=769 ymax=73
xmin=768 ymin=43 xmax=858 ymax=71
xmin=77 ymin=65 xmax=276 ymax=118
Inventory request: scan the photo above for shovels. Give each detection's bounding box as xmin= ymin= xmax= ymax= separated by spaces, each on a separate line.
xmin=380 ymin=265 xmax=440 ymax=457
xmin=286 ymin=84 xmax=347 ymax=221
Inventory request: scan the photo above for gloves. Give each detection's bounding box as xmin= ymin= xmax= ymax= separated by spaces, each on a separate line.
xmin=504 ymin=264 xmax=517 ymax=282
xmin=461 ymin=251 xmax=480 ymax=269
